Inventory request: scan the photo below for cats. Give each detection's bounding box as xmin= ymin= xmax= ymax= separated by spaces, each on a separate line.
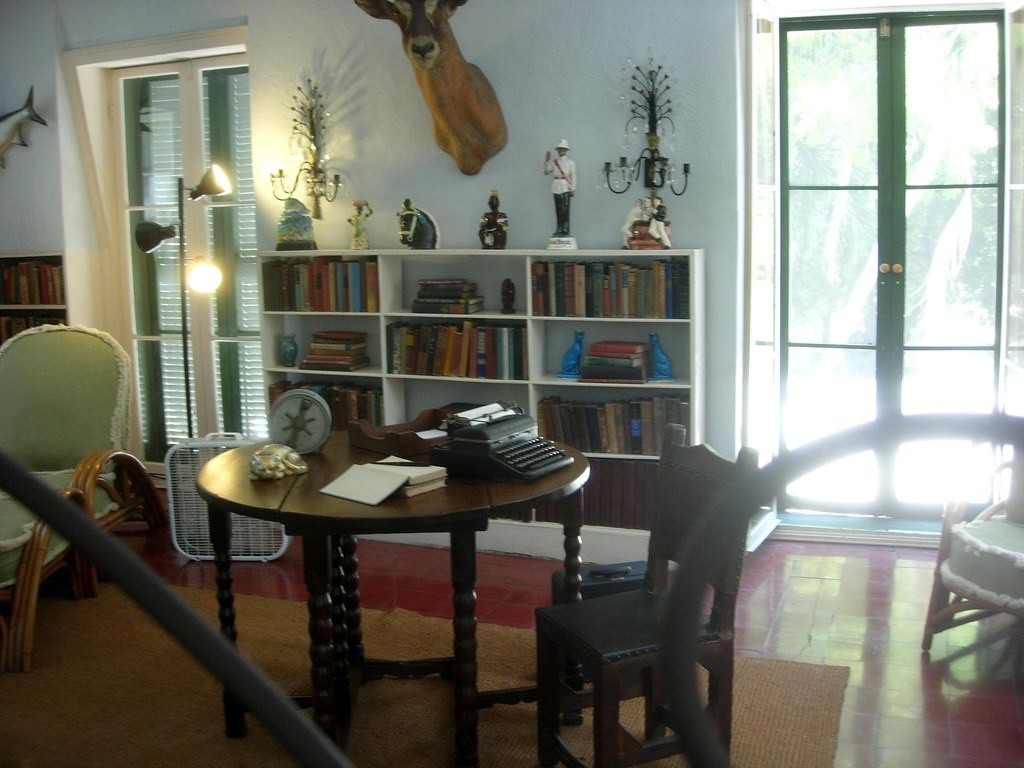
xmin=248 ymin=443 xmax=308 ymax=482
xmin=647 ymin=331 xmax=673 ymax=382
xmin=560 ymin=331 xmax=585 ymax=375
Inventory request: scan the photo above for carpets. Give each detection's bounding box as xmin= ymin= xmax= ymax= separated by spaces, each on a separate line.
xmin=2 ymin=583 xmax=851 ymax=767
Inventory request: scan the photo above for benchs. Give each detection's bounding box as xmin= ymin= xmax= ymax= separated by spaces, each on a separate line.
xmin=1 ymin=322 xmax=168 ymax=671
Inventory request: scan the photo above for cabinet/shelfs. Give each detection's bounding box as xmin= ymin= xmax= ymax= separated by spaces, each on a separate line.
xmin=256 ymin=249 xmax=704 ymax=536
xmin=0 ymin=254 xmax=66 ymax=311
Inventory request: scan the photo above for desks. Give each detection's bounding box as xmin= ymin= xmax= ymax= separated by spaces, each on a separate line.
xmin=195 ymin=426 xmax=592 ymax=765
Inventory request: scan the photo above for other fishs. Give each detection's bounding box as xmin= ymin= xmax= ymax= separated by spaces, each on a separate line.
xmin=1 ymin=84 xmax=50 ymax=170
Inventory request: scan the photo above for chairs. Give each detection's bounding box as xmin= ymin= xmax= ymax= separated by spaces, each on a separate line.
xmin=532 ymin=423 xmax=762 ymax=765
xmin=920 ymin=440 xmax=1023 ymax=692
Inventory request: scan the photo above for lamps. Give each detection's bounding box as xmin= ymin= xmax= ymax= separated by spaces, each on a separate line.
xmin=134 ymin=163 xmax=234 ymax=439
xmin=596 ymin=44 xmax=695 ymax=250
xmin=269 ymin=76 xmax=345 ymax=220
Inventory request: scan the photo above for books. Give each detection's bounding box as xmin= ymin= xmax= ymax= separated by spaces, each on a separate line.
xmin=385 ymin=320 xmax=529 ymax=381
xmin=261 ymin=256 xmax=380 ymax=312
xmin=390 ymin=476 xmax=447 ymax=498
xmin=411 ymin=279 xmax=484 ymax=315
xmin=537 ymin=395 xmax=690 ymax=457
xmin=0 ymin=258 xmax=67 ymax=347
xmin=267 ymin=380 xmax=384 ymax=431
xmin=575 ymin=341 xmax=654 ymax=385
xmin=531 ymin=259 xmax=690 ymax=320
xmin=318 ymin=454 xmax=448 ymax=507
xmin=299 ymin=330 xmax=370 ymax=373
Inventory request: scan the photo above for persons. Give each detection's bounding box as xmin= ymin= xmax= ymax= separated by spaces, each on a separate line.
xmin=395 ymin=197 xmax=420 ymax=244
xmin=347 ymin=200 xmax=375 ymax=250
xmin=621 ymin=194 xmax=672 ymax=250
xmin=478 ymin=194 xmax=508 ymax=250
xmin=544 ymin=137 xmax=577 ymax=237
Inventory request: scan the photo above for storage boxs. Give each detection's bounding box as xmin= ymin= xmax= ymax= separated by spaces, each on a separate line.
xmin=550 ymin=560 xmax=648 ymax=606
xmin=164 ymin=438 xmax=295 ymax=563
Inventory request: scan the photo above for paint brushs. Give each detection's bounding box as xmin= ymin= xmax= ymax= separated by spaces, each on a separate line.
xmin=372 ymin=461 xmax=430 ymax=467
